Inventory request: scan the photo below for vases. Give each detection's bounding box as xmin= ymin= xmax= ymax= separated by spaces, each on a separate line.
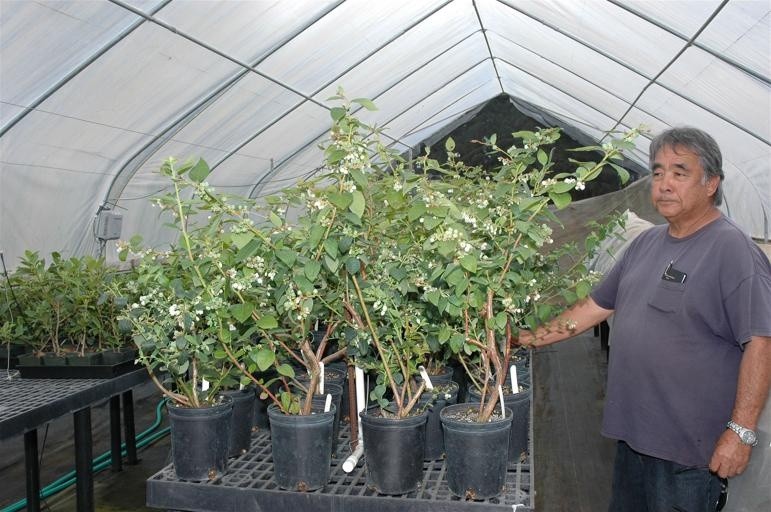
xmin=166 ymin=337 xmax=531 ymax=501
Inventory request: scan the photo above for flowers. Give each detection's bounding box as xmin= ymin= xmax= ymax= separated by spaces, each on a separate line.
xmin=0 ymin=88 xmax=651 ymax=424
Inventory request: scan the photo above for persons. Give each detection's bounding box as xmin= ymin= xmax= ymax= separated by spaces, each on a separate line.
xmin=518 ymin=127 xmax=771 ymax=512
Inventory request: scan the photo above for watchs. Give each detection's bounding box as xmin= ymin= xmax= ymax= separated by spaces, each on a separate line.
xmin=727 ymin=420 xmax=758 ymax=447
